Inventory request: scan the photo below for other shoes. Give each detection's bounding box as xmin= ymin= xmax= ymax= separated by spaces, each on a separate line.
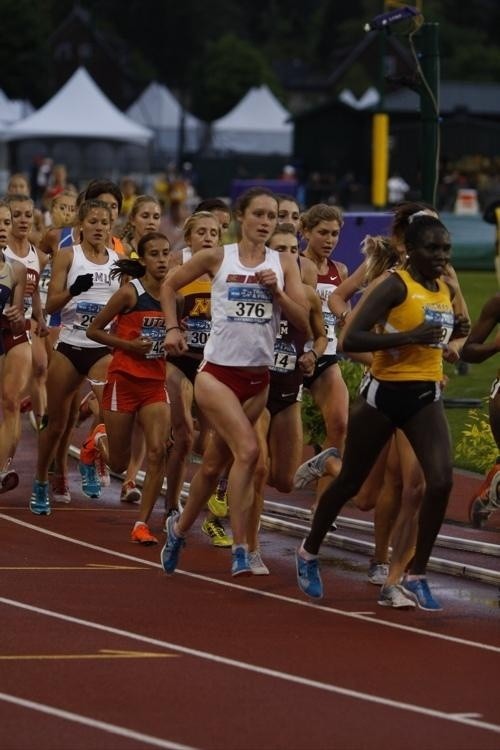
xmin=2 ymin=468 xmax=19 ymax=493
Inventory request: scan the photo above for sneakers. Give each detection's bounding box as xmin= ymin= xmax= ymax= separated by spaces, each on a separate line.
xmin=399 ymin=574 xmax=442 ymax=612
xmin=51 ymin=475 xmax=71 ymax=504
xmin=294 ymin=547 xmax=324 ymax=600
xmin=294 ymin=444 xmax=343 ymax=490
xmin=366 ymin=556 xmax=391 ymax=583
xmin=28 ymin=474 xmax=52 ymax=516
xmin=378 ymin=583 xmax=416 ymax=608
xmin=78 ymin=424 xmax=111 ymax=499
xmin=121 ymin=480 xmax=268 ymax=577
xmin=311 ymin=505 xmax=337 ymax=533
xmin=468 ymin=464 xmax=500 ymax=530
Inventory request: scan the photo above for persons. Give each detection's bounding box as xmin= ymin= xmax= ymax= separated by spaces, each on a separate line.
xmin=295 ymin=215 xmax=472 ymax=611
xmin=386 ymin=156 xmax=500 ymax=223
xmin=460 ymin=297 xmax=500 ymax=528
xmin=0 ymin=147 xmax=124 ymax=517
xmin=292 ymin=203 xmax=456 ymax=584
xmin=120 ymin=165 xmax=362 ymax=225
xmin=86 ymin=187 xmax=352 ymax=577
xmin=337 ymin=208 xmax=472 ymax=614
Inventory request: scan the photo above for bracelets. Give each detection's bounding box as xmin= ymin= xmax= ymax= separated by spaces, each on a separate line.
xmin=341 ymin=311 xmax=348 ymax=319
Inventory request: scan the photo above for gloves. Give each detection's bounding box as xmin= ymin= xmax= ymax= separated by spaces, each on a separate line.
xmin=70 ymin=274 xmax=93 ymax=296
